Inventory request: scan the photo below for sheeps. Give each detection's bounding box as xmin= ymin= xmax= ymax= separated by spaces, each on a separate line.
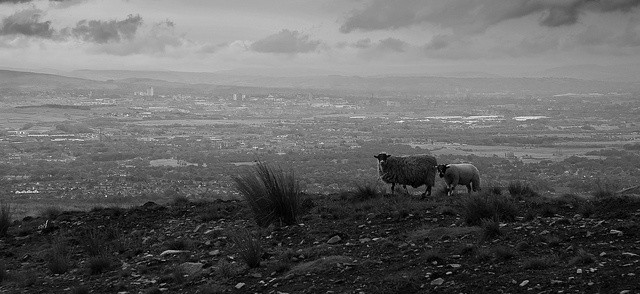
xmin=436 ymin=162 xmax=480 ymax=197
xmin=373 ymin=151 xmax=438 ymax=196
